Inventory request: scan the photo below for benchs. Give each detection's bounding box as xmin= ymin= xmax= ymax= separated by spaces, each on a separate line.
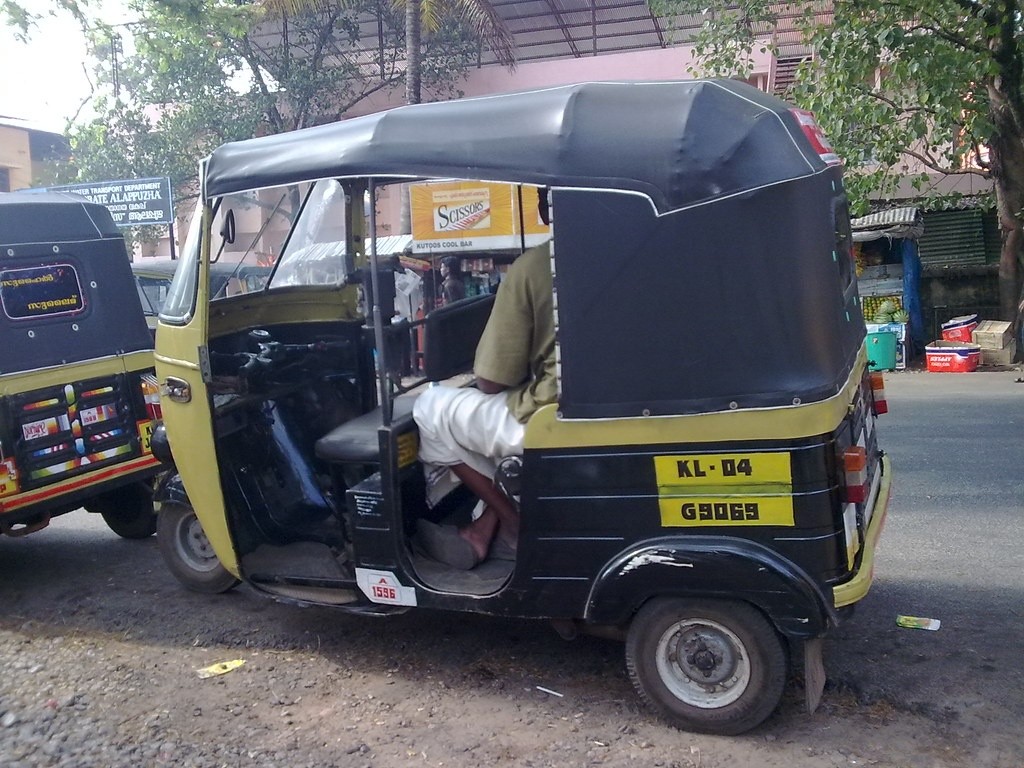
xmin=313 ymin=292 xmax=499 ymax=471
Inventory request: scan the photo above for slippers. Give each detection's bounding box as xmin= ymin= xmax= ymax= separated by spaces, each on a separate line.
xmin=417 ymin=518 xmax=478 ymax=570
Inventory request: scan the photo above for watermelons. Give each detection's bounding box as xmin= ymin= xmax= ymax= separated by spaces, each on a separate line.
xmin=875 ymin=299 xmax=910 ymax=325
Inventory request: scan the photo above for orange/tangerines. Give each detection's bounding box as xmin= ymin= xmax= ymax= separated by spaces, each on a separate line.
xmin=864 ymin=294 xmax=902 ymax=323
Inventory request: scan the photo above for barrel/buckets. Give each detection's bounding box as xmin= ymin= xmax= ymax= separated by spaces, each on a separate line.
xmin=866 ymin=331 xmax=897 ymax=371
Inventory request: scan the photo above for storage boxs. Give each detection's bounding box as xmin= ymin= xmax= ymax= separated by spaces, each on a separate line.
xmin=866 ymin=307 xmax=1020 ymax=375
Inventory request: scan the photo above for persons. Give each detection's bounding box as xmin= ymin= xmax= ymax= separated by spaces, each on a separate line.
xmin=438 ymin=253 xmax=466 ymax=307
xmin=410 ymin=180 xmax=556 ymax=573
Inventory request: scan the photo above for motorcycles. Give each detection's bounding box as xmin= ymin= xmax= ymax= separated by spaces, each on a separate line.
xmin=118 ymin=253 xmax=272 ymax=351
xmin=143 ymin=82 xmax=894 ymax=743
xmin=1 ymin=190 xmax=185 ymax=565
